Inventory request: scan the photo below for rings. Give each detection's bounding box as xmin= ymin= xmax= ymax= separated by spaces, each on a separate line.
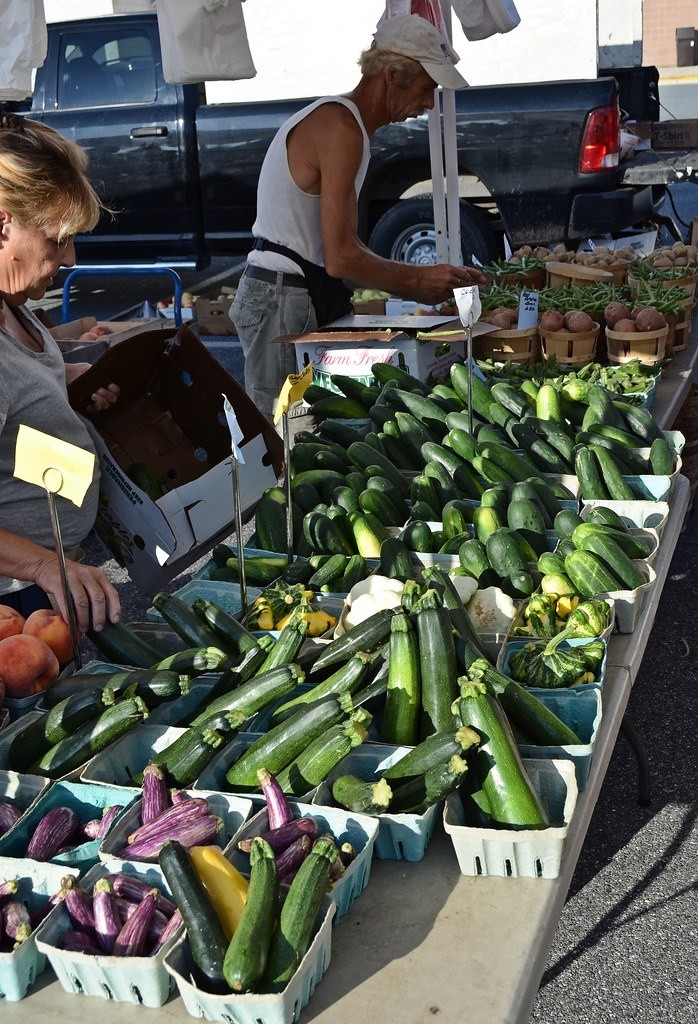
xmin=459 ymin=279 xmax=460 ymax=283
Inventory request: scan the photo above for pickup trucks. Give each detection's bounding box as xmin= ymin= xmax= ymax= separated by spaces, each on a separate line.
xmin=1 ymin=10 xmax=656 ymax=309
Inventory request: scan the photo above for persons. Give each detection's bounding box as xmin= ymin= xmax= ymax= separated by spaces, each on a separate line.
xmin=230 ymin=12 xmax=489 ymax=428
xmin=0 ymin=107 xmax=123 ymax=637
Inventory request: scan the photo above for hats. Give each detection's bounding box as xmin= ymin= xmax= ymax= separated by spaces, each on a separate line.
xmin=374 ymin=13 xmax=471 ymax=89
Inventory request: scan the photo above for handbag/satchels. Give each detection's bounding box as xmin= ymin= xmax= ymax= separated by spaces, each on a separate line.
xmin=304 ymin=259 xmax=354 ymax=327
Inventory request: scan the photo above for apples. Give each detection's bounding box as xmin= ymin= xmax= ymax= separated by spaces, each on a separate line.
xmin=349 ymin=289 xmax=390 ymax=303
xmin=155 ymin=291 xmax=197 ymax=308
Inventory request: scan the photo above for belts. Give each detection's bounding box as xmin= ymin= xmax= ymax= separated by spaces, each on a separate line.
xmin=245 ymin=265 xmax=308 ymax=288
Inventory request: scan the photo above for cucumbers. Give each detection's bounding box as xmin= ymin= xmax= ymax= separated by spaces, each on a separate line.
xmin=158 ymin=832 xmax=340 ymax=994
xmin=38 ymin=365 xmax=668 ymax=850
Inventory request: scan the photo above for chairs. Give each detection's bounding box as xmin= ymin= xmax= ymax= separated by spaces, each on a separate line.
xmin=108 ymin=62 xmax=139 ymax=86
xmin=63 ymin=54 xmax=104 ymax=102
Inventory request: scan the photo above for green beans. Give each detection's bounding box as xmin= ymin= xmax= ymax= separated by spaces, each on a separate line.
xmin=445 ymin=251 xmax=698 ymax=315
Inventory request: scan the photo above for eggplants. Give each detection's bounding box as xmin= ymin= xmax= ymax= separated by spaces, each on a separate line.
xmin=0 ymin=763 xmax=352 ymax=953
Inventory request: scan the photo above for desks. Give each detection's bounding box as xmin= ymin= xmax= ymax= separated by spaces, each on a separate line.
xmin=631 ymin=344 xmax=695 ymax=436
xmin=1 ymin=482 xmax=689 ymax=1024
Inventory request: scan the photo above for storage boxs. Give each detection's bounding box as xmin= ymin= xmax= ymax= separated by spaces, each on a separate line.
xmin=625 ymin=121 xmax=651 ymax=139
xmin=0 ymin=308 xmax=665 ymax=1024
xmin=651 ymin=118 xmax=697 ymax=149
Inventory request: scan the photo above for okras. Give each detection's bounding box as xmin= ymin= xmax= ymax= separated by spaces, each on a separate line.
xmin=462 ymin=353 xmax=654 ymax=395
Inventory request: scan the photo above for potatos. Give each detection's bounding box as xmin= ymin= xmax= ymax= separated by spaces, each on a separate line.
xmin=507 ymin=241 xmax=698 ymax=267
xmin=414 ymin=302 xmax=667 ymax=332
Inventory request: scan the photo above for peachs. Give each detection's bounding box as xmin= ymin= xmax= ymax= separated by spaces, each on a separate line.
xmin=0 ymin=604 xmax=73 ymax=706
